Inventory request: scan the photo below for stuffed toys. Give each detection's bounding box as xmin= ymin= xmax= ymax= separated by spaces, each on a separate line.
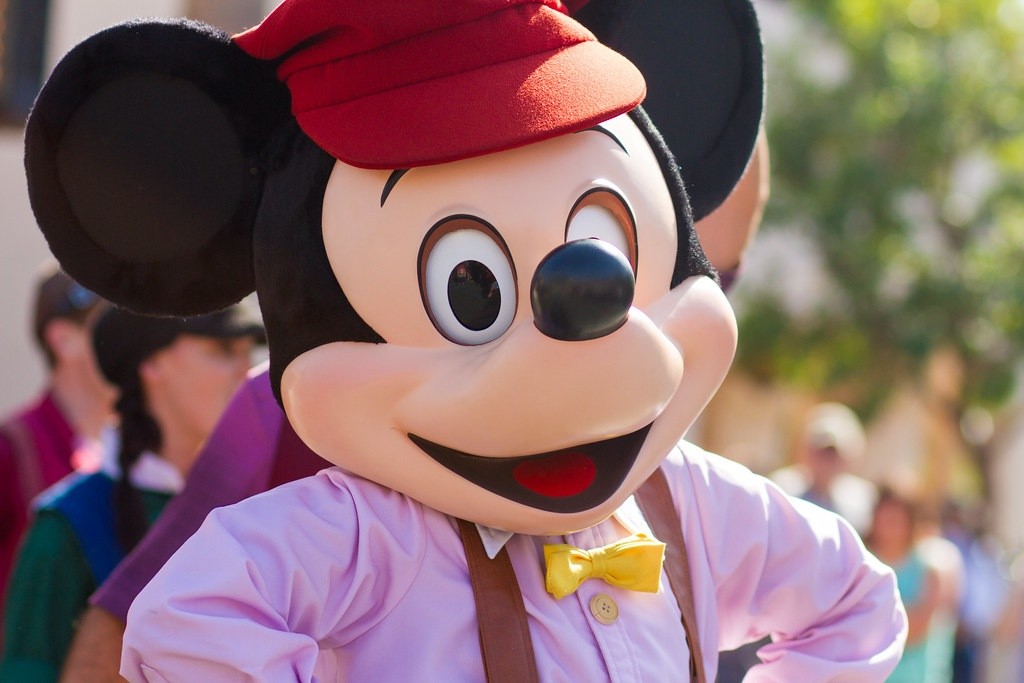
xmin=24 ymin=0 xmax=908 ymax=683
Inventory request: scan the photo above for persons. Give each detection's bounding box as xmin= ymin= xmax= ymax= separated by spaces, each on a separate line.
xmin=0 ymin=268 xmax=331 ymax=683
xmin=761 ymin=398 xmax=1024 ymax=683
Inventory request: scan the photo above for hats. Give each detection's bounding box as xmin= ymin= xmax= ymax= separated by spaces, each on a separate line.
xmin=802 ymin=401 xmax=864 ymax=461
xmin=93 ymin=300 xmax=269 ymax=384
